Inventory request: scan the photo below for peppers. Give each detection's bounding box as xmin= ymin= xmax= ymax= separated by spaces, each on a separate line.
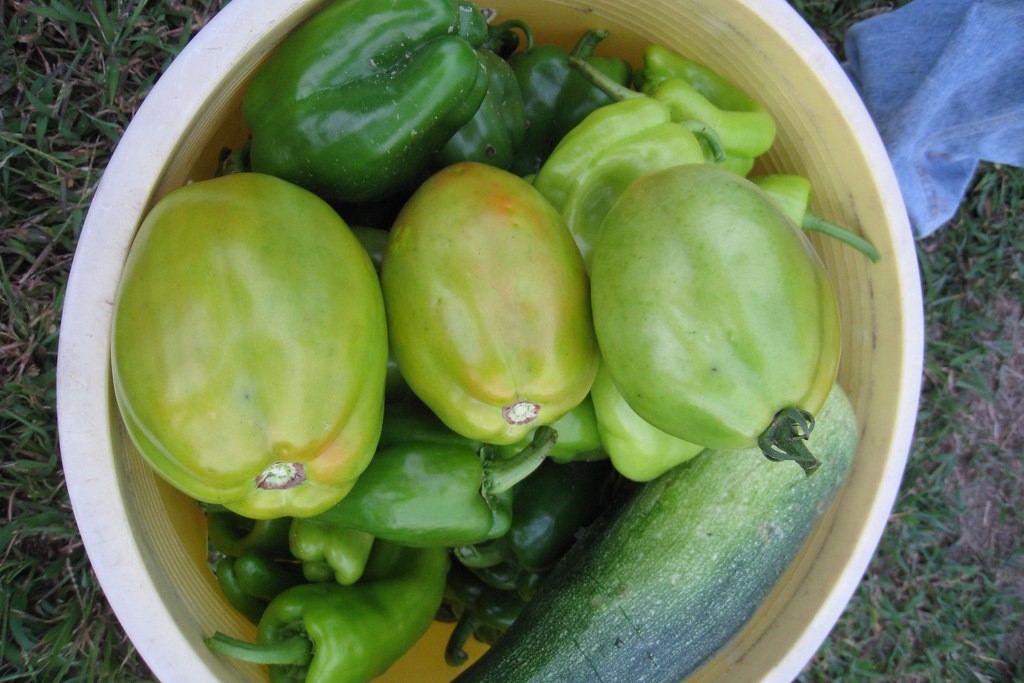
xmin=110 ymin=0 xmax=880 ymax=683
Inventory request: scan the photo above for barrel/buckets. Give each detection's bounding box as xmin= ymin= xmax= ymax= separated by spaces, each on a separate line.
xmin=57 ymin=0 xmax=923 ymax=683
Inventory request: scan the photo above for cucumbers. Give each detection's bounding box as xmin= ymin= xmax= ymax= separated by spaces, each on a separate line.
xmin=448 ymin=382 xmax=858 ymax=683
